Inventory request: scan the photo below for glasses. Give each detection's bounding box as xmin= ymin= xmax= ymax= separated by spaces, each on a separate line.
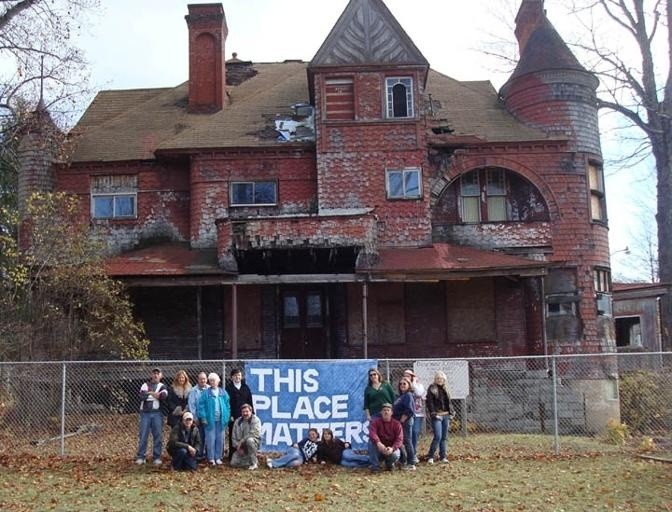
xmin=399 ymin=383 xmax=407 ymax=385
xmin=370 ymin=373 xmax=376 ymax=376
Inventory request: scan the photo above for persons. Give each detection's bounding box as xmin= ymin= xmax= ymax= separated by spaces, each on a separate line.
xmin=425 ymin=370 xmax=455 ymax=465
xmin=402 ymin=368 xmax=427 ymax=465
xmin=362 ymin=368 xmax=399 ymax=421
xmin=367 ymin=402 xmax=404 ymax=475
xmin=318 ymin=428 xmax=369 ymax=468
xmin=134 ymin=367 xmax=263 ymax=474
xmin=264 ymin=428 xmax=320 ymax=469
xmin=392 ymin=377 xmax=417 ymax=471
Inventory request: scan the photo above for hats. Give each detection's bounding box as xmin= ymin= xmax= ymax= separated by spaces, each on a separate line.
xmin=404 ymin=370 xmax=416 ymax=377
xmin=382 ymin=403 xmax=392 ymax=408
xmin=183 ymin=412 xmax=193 ymax=420
xmin=152 ymin=367 xmax=161 ymax=373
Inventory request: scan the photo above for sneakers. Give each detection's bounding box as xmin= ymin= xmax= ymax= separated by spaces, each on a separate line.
xmin=216 ymin=459 xmax=223 ymax=464
xmin=427 ymin=458 xmax=433 ymax=463
xmin=153 ymin=459 xmax=162 ymax=465
xmin=267 ymin=458 xmax=272 ymax=468
xmin=400 ymin=464 xmax=416 ymax=472
xmin=439 ymin=458 xmax=449 ymax=464
xmin=209 ymin=460 xmax=216 ymax=466
xmin=135 ymin=459 xmax=146 ymax=465
xmin=387 ymin=463 xmax=394 ymax=470
xmin=249 ymin=457 xmax=258 ymax=470
xmin=371 ymin=469 xmax=380 ymax=473
